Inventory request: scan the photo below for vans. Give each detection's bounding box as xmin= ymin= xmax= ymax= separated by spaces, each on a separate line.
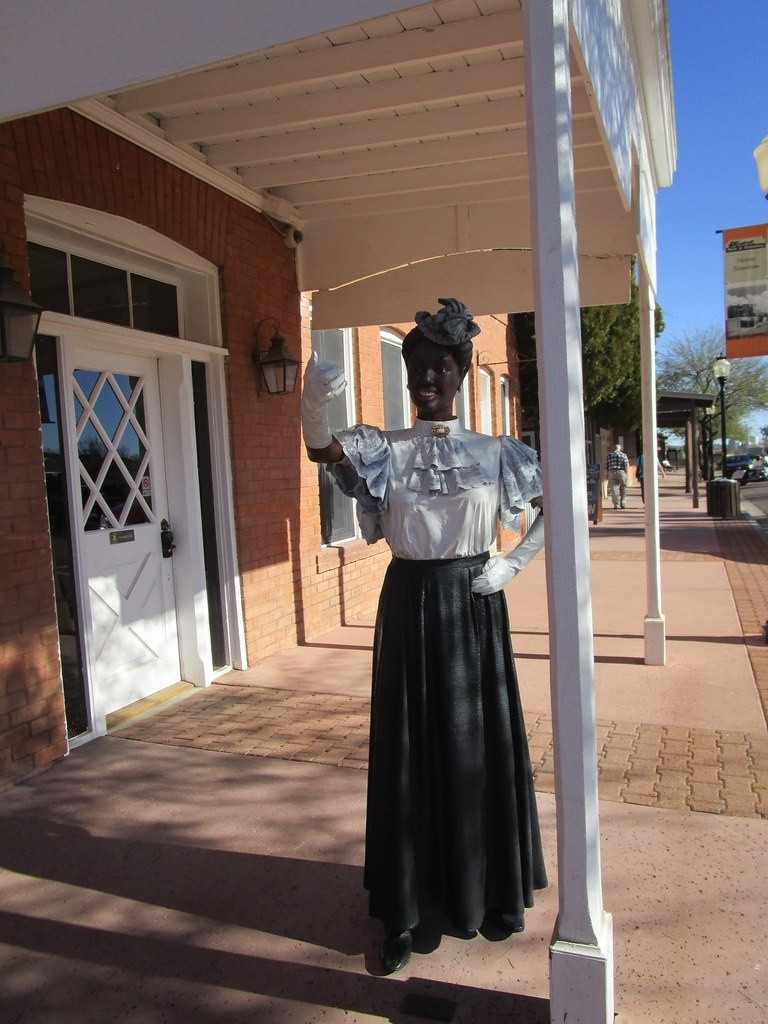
xmin=745 ymin=446 xmax=768 ymax=480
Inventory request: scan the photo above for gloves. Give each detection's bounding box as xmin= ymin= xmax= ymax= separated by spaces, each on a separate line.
xmin=301 ymin=351 xmax=347 ymax=449
xmin=471 ymin=515 xmax=545 ymax=595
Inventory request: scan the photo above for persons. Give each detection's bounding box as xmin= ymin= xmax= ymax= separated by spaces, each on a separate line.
xmin=636 ymin=453 xmax=666 ymax=509
xmin=662 ymin=457 xmax=677 ymax=472
xmin=604 ymin=444 xmax=629 ymax=509
xmin=300 ymin=297 xmax=546 ymax=971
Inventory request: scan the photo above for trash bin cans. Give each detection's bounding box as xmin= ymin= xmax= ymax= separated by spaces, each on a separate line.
xmin=706 ymin=479 xmax=741 ymax=520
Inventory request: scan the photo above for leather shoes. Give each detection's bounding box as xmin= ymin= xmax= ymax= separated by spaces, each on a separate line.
xmin=378 ymin=928 xmax=412 ymax=974
xmin=497 ymin=913 xmax=525 ymax=932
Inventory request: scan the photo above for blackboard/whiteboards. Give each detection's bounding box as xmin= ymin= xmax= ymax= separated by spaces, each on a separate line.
xmin=587 ymin=463 xmax=603 ymax=519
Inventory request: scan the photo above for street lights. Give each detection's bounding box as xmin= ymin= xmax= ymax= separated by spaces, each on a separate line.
xmin=713 ymin=351 xmax=732 ymax=477
xmin=705 ymin=404 xmax=717 ymax=478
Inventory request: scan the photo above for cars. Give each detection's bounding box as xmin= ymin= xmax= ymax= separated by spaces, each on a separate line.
xmin=722 ymin=454 xmax=762 ymax=485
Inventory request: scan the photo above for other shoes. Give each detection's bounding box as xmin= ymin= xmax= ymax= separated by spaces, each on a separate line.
xmin=614 ymin=507 xmax=618 ymax=510
xmin=621 ymin=504 xmax=625 ymax=508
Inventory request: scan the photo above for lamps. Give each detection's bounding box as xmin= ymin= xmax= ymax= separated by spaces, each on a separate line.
xmin=0 ymin=238 xmax=49 ymax=361
xmin=251 ymin=317 xmax=304 ymax=398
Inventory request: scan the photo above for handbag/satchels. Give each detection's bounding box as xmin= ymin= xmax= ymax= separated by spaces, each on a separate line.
xmin=636 ymin=455 xmax=642 ymax=478
